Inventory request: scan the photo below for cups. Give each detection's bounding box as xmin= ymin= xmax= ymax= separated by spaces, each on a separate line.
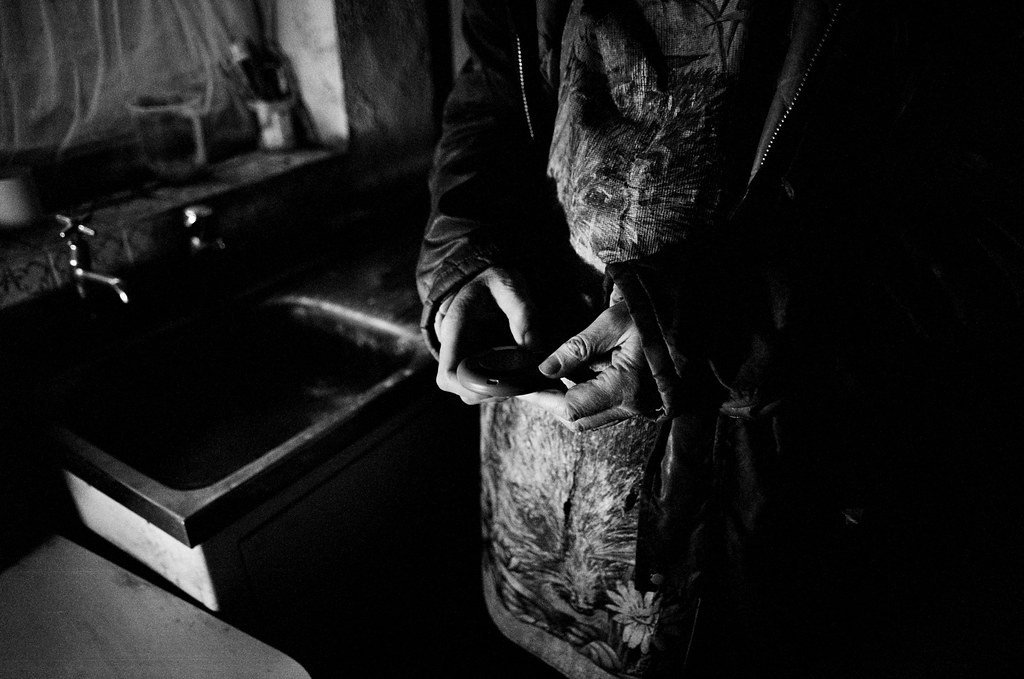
xmin=127 ymin=92 xmax=205 ymax=179
xmin=246 ymin=90 xmax=293 ymax=148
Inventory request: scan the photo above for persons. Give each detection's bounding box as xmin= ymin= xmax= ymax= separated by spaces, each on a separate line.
xmin=414 ymin=0 xmax=1024 ymax=679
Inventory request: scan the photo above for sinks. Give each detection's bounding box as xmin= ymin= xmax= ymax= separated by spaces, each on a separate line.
xmin=19 ymin=295 xmax=477 ymax=619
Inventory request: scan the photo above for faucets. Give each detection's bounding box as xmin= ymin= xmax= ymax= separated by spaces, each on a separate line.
xmin=55 ymin=209 xmax=133 ymax=307
xmin=183 ymin=201 xmax=228 ymax=259
